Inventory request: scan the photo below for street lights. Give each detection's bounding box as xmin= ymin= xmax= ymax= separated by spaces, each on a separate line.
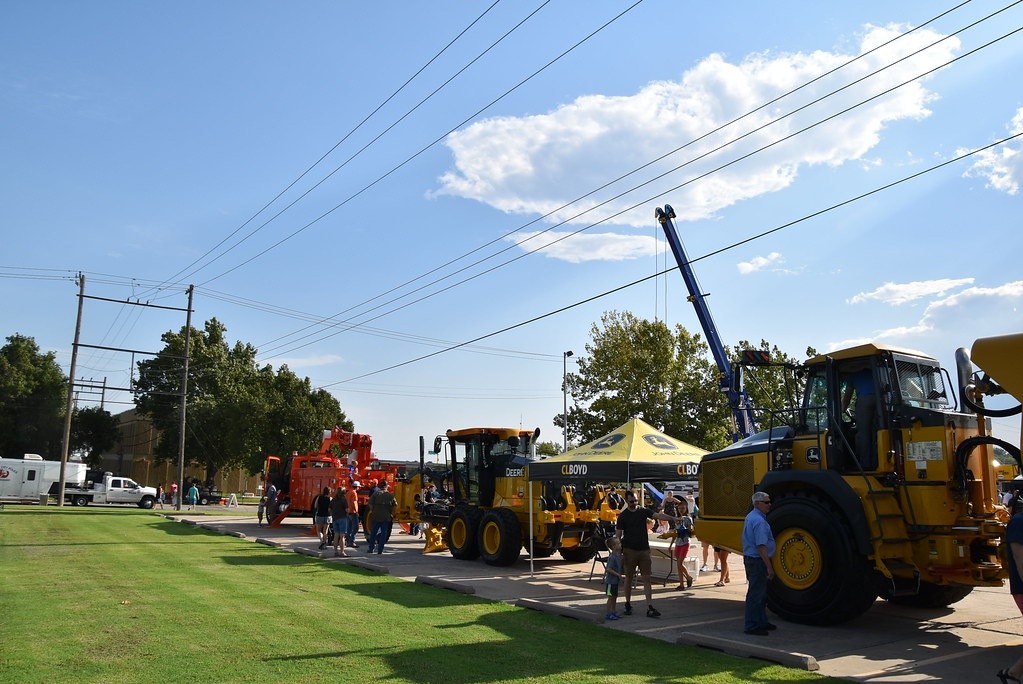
xmin=564 ymin=351 xmax=573 ymax=452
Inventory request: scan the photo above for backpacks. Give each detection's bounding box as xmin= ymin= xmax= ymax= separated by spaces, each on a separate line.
xmin=610 ymin=497 xmax=617 ymax=509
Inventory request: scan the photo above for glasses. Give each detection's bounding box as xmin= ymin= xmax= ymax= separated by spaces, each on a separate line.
xmin=626 ymin=499 xmax=639 ymax=503
xmin=759 ymin=500 xmax=771 ymax=504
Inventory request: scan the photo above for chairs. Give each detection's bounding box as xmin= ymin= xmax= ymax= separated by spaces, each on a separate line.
xmin=589 ymin=538 xmax=611 ymax=584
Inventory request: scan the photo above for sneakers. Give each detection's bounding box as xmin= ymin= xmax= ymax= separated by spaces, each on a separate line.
xmin=700 ymin=565 xmax=709 ymax=572
xmin=714 ymin=565 xmax=721 ymax=572
xmin=724 ymin=578 xmax=730 ymax=583
xmin=714 ymin=581 xmax=725 ymax=586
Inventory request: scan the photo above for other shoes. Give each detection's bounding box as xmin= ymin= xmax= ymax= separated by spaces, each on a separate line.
xmin=319 ymin=543 xmax=359 ymax=557
xmin=624 ymin=601 xmax=632 ymax=616
xmin=744 ymin=623 xmax=777 ymax=636
xmin=687 ymin=577 xmax=692 ymax=588
xmin=605 ymin=611 xmax=623 ymax=620
xmin=419 ymin=537 xmax=422 ymax=539
xmin=647 ymin=608 xmax=661 ymax=617
xmin=367 ymin=549 xmax=374 ymax=553
xmin=673 ymin=586 xmax=685 ymax=591
xmin=378 ymin=552 xmax=382 ymax=554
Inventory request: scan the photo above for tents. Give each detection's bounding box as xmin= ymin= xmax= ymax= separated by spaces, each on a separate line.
xmin=528 ymin=417 xmax=713 ymax=578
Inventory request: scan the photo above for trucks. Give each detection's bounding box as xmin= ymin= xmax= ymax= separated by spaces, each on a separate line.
xmin=0 ymin=454 xmax=160 ymax=509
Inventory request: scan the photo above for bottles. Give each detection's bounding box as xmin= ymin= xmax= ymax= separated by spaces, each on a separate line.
xmin=840 ymin=411 xmax=851 ymax=422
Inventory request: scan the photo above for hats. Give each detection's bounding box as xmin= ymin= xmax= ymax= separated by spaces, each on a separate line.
xmin=353 ymin=481 xmax=361 ymax=486
xmin=373 ymin=479 xmax=378 ymax=484
xmin=610 ymin=487 xmax=616 ymax=491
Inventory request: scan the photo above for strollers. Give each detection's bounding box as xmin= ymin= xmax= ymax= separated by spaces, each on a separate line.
xmin=327 ymin=523 xmax=340 ymax=545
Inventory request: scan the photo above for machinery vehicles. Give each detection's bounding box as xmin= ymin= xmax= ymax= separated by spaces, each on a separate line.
xmin=262 ymin=425 xmax=412 ymax=536
xmin=393 ymin=428 xmax=621 ymax=566
xmin=694 ymin=334 xmax=1023 ymax=624
xmin=655 ymin=204 xmax=758 ymax=444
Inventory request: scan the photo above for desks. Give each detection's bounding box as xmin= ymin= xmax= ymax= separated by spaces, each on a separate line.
xmin=649 ymin=541 xmax=698 ymax=587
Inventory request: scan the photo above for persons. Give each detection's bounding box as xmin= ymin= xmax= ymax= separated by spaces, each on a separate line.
xmin=700 ymin=541 xmax=721 ymax=572
xmin=263 ymin=479 xmax=285 ymax=525
xmin=842 ymin=370 xmax=887 ymax=471
xmin=257 ymin=496 xmax=267 ymax=528
xmin=743 ymin=491 xmax=778 ymax=636
xmin=668 ymin=501 xmax=693 ymax=590
xmin=714 ymin=545 xmax=731 ymax=586
xmin=348 ymin=461 xmax=359 ymax=483
xmin=170 ymin=481 xmax=178 ymax=507
xmin=187 ymin=483 xmax=199 ymax=510
xmin=609 ymin=487 xmax=621 ymax=525
xmin=408 ymin=485 xmax=452 ymax=539
xmin=154 ymin=483 xmax=164 ymax=511
xmin=616 ymin=490 xmax=685 ymax=617
xmin=647 ymin=490 xmax=695 ymax=533
xmin=997 ymin=489 xmax=1013 ymax=506
xmin=606 ymin=537 xmax=626 ymax=620
xmin=314 ymin=478 xmax=398 ymax=557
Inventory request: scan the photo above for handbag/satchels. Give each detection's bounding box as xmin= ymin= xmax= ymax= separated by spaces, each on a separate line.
xmin=618 ymin=498 xmax=625 ymax=510
xmin=694 ymin=503 xmax=699 ymax=516
